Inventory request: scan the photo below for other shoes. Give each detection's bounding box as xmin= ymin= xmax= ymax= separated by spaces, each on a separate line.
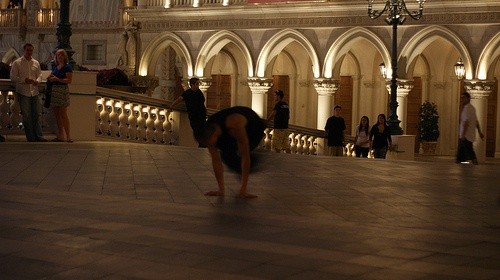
xmin=67 ymin=138 xmax=73 ymax=142
xmin=52 ymin=137 xmax=65 ymax=142
xmin=27 ymin=138 xmax=48 ymax=142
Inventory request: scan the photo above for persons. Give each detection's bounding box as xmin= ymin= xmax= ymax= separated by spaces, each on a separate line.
xmin=456 ymin=92 xmax=485 ymax=165
xmin=195 ymin=107 xmax=264 ymax=199
xmin=10 ymin=43 xmax=48 ymax=142
xmin=369 ymin=114 xmax=393 ymax=159
xmin=267 ymin=89 xmax=291 ymax=154
xmin=354 ymin=116 xmax=372 ymax=157
xmin=47 ymin=49 xmax=74 ymax=142
xmin=170 ymin=77 xmax=208 ymax=148
xmin=325 ymin=106 xmax=346 ymax=156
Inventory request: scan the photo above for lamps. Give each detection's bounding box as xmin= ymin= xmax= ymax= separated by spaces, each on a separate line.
xmin=454 ymin=58 xmax=468 ymax=81
xmin=379 ymin=62 xmax=389 ymax=78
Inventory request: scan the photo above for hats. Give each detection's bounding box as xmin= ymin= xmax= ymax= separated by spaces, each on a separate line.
xmin=274 ymin=90 xmax=284 ymax=97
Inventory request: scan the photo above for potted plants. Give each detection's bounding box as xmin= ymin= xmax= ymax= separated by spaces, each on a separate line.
xmin=417 ymin=99 xmax=442 ymax=154
